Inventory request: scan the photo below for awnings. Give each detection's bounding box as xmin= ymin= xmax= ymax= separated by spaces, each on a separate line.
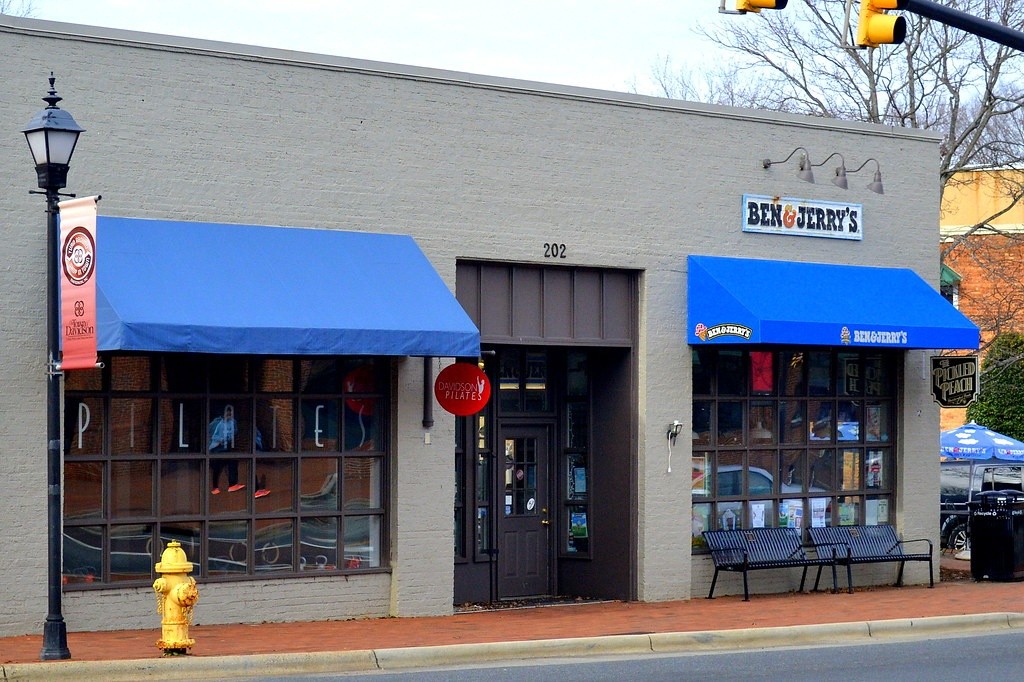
xmin=56 ymin=213 xmax=480 ymax=357
xmin=687 ymin=255 xmax=980 ymax=347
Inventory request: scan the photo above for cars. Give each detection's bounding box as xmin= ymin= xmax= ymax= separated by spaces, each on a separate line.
xmin=691 ymin=463 xmax=834 ymax=534
xmin=789 ymin=420 xmax=880 ymax=489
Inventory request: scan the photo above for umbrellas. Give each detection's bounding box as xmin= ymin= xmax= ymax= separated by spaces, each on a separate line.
xmin=940 ymin=419 xmax=1024 ymax=559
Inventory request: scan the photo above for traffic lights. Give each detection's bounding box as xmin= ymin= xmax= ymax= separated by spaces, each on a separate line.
xmin=735 ymin=0 xmax=789 ymax=13
xmin=856 ymin=0 xmax=907 ymax=48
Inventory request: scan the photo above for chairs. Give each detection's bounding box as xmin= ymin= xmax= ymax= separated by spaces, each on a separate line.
xmin=941 ymin=499 xmax=966 ymax=554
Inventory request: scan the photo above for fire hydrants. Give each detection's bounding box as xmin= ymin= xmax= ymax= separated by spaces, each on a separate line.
xmin=152 ymin=537 xmax=197 ymax=657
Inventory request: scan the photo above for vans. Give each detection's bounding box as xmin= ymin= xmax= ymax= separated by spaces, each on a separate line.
xmin=939 ymin=459 xmax=1024 ymax=550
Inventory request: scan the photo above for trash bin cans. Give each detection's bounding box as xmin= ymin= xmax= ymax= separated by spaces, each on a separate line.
xmin=968 ymin=488 xmax=1024 ymax=583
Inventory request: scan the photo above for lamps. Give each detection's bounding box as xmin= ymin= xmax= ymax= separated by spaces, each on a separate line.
xmin=800 ymin=152 xmax=848 ymax=190
xmin=762 ymin=146 xmax=815 ymax=184
xmin=836 ymin=158 xmax=884 ymax=194
xmin=667 ymin=419 xmax=682 ymax=439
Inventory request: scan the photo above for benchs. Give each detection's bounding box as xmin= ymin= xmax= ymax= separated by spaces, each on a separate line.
xmin=807 ymin=525 xmax=934 ymax=595
xmin=701 ymin=527 xmax=839 ymax=602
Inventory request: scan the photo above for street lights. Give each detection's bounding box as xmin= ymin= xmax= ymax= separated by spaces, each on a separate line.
xmin=16 ymin=68 xmax=88 ymax=660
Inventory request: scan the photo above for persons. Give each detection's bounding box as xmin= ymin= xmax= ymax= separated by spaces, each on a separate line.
xmin=254 ymin=426 xmax=269 ymax=498
xmin=207 ymin=406 xmax=245 ymax=494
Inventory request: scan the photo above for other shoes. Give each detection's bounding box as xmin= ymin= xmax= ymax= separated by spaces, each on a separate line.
xmin=211 ymin=488 xmax=220 ymax=494
xmin=228 ymin=484 xmax=245 ymax=492
xmin=255 ymin=489 xmax=270 ymax=498
